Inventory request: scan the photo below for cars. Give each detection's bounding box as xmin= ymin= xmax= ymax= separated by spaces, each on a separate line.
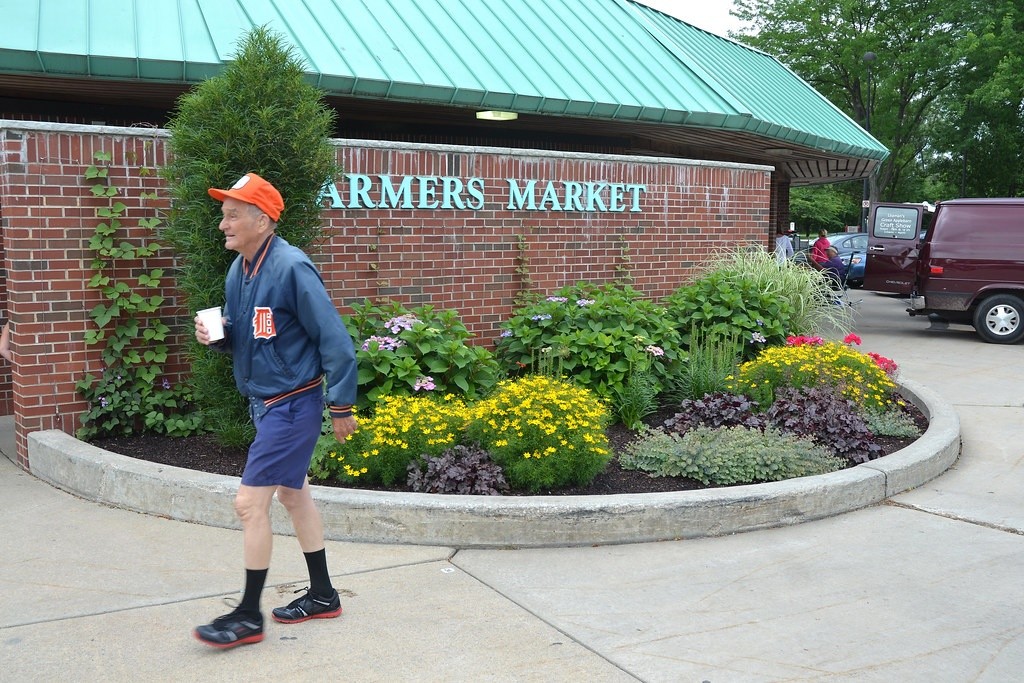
xmin=808 ymin=232 xmax=868 ymax=289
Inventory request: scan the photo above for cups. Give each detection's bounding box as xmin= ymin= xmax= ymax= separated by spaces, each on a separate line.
xmin=195 ymin=306 xmax=225 ymax=341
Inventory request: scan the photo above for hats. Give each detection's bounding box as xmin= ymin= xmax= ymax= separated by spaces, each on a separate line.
xmin=207 ymin=172 xmax=285 ymax=223
xmin=824 ymin=246 xmax=838 ymax=254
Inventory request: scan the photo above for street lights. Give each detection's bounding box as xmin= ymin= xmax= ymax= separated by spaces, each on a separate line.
xmin=864 ymin=52 xmax=879 ymax=132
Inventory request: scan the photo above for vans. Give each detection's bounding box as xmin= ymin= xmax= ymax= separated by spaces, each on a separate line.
xmin=863 ymin=197 xmax=1024 ymax=345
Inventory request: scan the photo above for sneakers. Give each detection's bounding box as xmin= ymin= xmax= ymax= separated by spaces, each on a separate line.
xmin=196 ymin=607 xmax=266 ymax=648
xmin=273 ymin=591 xmax=341 ymax=624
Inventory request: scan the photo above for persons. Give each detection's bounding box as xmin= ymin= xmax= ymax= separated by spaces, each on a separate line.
xmin=776 ymin=233 xmax=793 ymax=269
xmin=0 ymin=321 xmax=11 ymax=363
xmin=194 ymin=173 xmax=363 ymax=649
xmin=810 ymin=246 xmax=845 ymax=291
xmin=813 ymin=229 xmax=830 ymax=264
